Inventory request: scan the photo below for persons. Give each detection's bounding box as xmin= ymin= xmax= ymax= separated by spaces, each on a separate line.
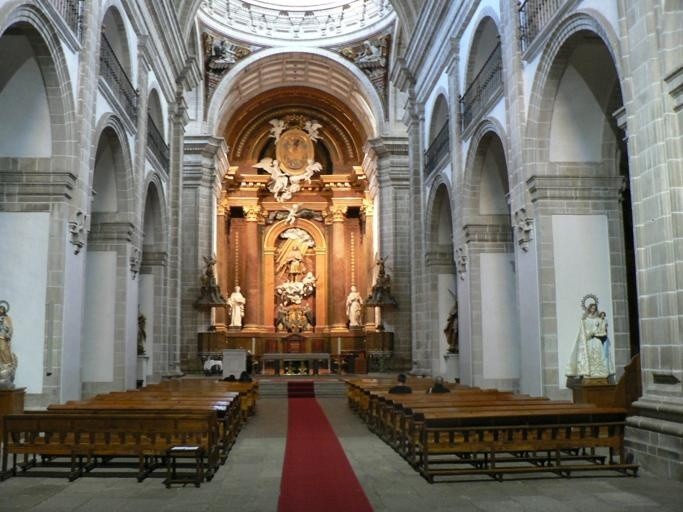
xmin=226 ymin=375 xmax=237 ymax=381
xmin=565 ymin=303 xmax=617 ymax=379
xmin=354 ymin=40 xmax=379 ymax=62
xmin=282 ymin=203 xmax=299 ymax=226
xmin=285 ymin=245 xmax=305 ymax=283
xmin=346 ymin=285 xmax=363 ymax=327
xmin=238 ymin=371 xmax=255 ymax=382
xmin=219 ymin=40 xmax=238 ymax=62
xmin=427 ymin=376 xmax=450 ymax=393
xmin=0 ymin=306 xmax=14 ymax=363
xmin=303 ymin=159 xmax=314 ymax=184
xmin=227 ymin=285 xmax=246 ymax=326
xmin=306 ymin=120 xmax=324 ymax=143
xmin=271 ymin=119 xmax=287 ymax=145
xmin=270 ymin=159 xmax=289 ymax=199
xmin=388 ymin=374 xmax=412 ymax=393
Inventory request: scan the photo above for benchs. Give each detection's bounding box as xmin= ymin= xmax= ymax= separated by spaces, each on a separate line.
xmin=1 ymin=379 xmax=258 ymax=490
xmin=347 ymin=375 xmax=624 ymax=485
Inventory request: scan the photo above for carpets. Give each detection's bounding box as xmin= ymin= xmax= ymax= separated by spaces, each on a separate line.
xmin=275 ymin=381 xmax=371 ymax=511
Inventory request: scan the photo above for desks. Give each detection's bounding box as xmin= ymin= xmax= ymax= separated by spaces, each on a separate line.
xmin=261 ymin=352 xmax=330 ymax=376
